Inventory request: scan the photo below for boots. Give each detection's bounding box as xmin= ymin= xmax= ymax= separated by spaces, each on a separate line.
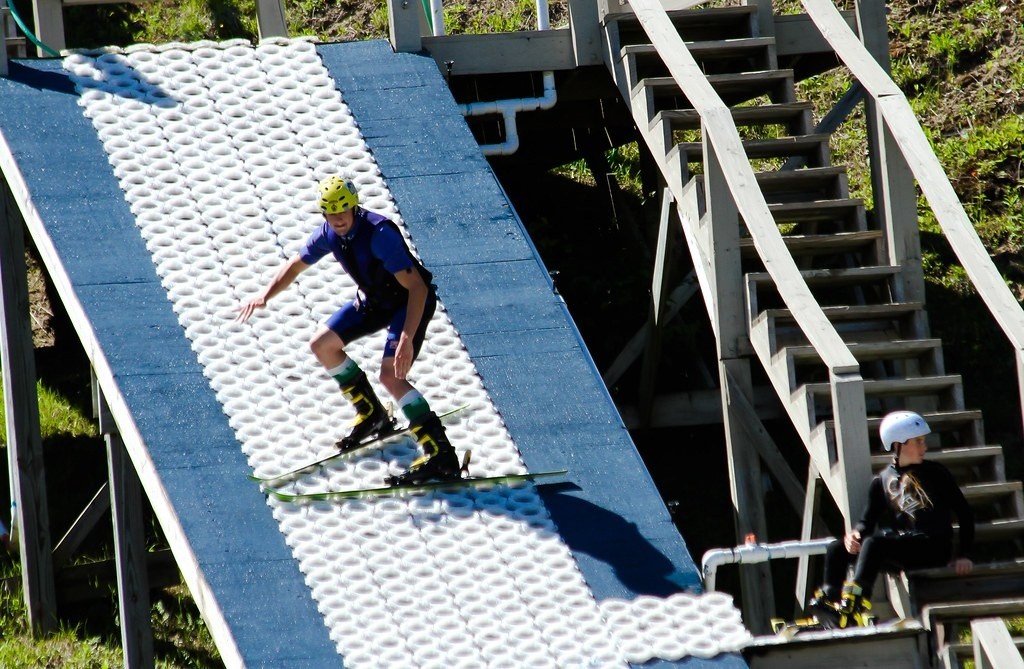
xmin=400 ymin=411 xmax=458 ymax=482
xmin=787 ymin=583 xmax=842 ymax=631
xmin=809 ymin=581 xmax=871 ymax=630
xmin=342 ymin=373 xmax=387 ymax=449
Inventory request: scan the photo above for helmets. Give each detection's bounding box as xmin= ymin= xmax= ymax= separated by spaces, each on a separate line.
xmin=317 ymin=175 xmax=359 ymax=214
xmin=879 ymin=411 xmax=931 ymax=452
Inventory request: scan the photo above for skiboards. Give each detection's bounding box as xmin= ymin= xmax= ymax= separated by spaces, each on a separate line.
xmin=262 ymin=468 xmax=569 ymax=503
xmin=245 ymin=403 xmax=472 ymax=482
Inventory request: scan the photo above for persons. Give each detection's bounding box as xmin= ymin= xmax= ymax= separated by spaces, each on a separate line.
xmin=231 ymin=176 xmax=460 ymax=486
xmin=788 ymin=410 xmax=977 ymax=632
